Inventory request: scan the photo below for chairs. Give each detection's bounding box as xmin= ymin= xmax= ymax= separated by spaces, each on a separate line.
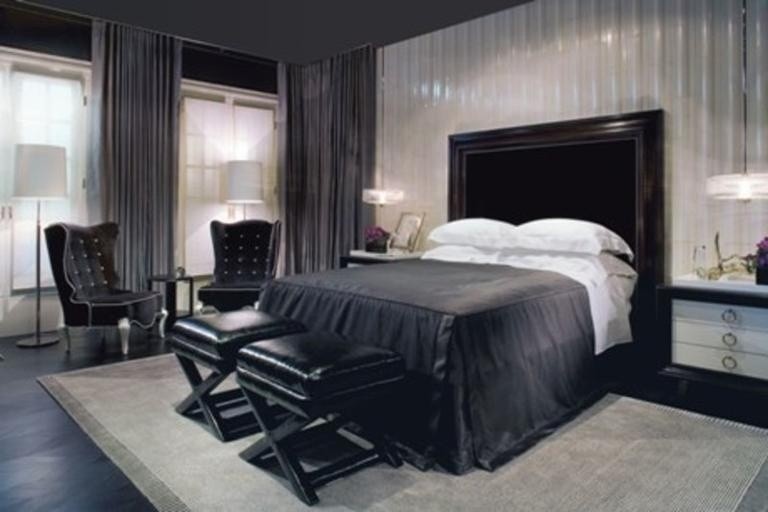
xmin=38 ymin=220 xmax=169 ymax=358
xmin=198 ymin=217 xmax=281 ymax=311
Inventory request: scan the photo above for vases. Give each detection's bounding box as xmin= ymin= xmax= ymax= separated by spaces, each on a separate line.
xmin=365 ymin=239 xmax=387 ymax=252
xmin=755 ymin=263 xmax=767 ymax=281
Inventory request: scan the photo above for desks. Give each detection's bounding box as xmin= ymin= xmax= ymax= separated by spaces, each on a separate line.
xmin=142 ymin=269 xmax=212 ymax=322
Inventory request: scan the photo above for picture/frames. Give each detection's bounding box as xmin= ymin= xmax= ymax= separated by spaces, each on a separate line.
xmin=389 ymin=212 xmax=425 ymax=254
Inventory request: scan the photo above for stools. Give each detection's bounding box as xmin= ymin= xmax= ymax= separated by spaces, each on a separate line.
xmin=166 ymin=308 xmax=308 ymax=442
xmin=234 ymin=335 xmax=408 ymax=505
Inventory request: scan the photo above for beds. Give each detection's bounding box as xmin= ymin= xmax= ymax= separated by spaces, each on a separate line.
xmin=260 ymin=105 xmax=662 ymax=477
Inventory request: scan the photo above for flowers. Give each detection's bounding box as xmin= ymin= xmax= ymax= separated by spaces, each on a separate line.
xmin=751 ymin=235 xmax=767 ymax=263
xmin=363 ymin=225 xmax=386 ymax=242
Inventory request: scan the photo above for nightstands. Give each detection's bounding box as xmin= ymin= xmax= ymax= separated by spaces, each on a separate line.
xmin=339 ymin=250 xmax=420 ymax=266
xmin=667 ymin=278 xmax=767 ymax=389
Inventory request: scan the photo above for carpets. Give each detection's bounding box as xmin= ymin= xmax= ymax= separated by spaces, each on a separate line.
xmin=35 ymin=354 xmax=765 ymax=512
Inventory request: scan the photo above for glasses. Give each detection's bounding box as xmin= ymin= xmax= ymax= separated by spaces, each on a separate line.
xmin=696 ymin=267 xmax=741 ymax=280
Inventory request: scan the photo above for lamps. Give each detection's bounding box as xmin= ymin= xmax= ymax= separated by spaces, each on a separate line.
xmin=11 ymin=144 xmax=68 ymax=350
xmin=353 ymin=2 xmax=402 ymax=208
xmin=702 ymin=2 xmax=767 ymax=206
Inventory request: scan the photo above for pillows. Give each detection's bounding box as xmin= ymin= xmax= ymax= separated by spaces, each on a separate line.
xmin=512 ymin=253 xmax=638 ymax=285
xmin=513 ymin=220 xmax=636 ymax=264
xmin=422 ymin=246 xmax=511 ymax=263
xmin=424 ymin=218 xmax=515 ymax=251
